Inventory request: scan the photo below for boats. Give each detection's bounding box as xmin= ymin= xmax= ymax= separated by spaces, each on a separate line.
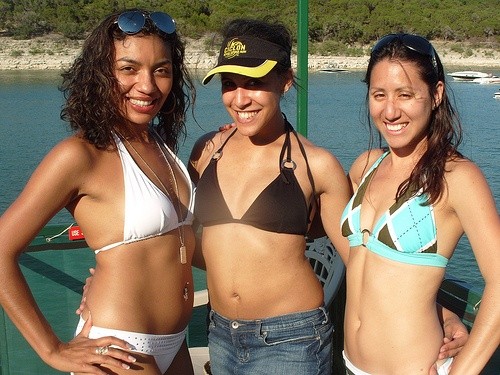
xmin=317 ymin=68 xmax=349 ymax=74
xmin=447 ymin=70 xmax=500 ymax=85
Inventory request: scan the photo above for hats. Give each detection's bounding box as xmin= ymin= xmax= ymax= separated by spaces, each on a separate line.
xmin=202 ymin=35 xmax=289 ymax=85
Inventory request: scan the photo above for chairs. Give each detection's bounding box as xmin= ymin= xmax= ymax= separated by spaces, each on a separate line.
xmin=304 ymin=233 xmax=345 ymax=309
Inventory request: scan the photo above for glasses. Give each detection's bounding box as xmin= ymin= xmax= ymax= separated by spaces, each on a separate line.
xmin=371 ymin=34 xmax=439 ymax=81
xmin=115 ymin=11 xmax=177 ymax=33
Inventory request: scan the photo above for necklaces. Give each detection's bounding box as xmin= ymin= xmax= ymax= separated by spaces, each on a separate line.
xmin=108 ymin=123 xmax=187 ymax=265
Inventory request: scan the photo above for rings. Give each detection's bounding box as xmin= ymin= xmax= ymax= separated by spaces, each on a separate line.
xmin=95 ymin=345 xmax=109 ymax=355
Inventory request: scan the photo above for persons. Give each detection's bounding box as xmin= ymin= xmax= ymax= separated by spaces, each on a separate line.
xmin=1 ymin=10 xmax=238 ymax=375
xmin=219 ymin=33 xmax=498 ymax=375
xmin=76 ymin=22 xmax=470 ymax=375
